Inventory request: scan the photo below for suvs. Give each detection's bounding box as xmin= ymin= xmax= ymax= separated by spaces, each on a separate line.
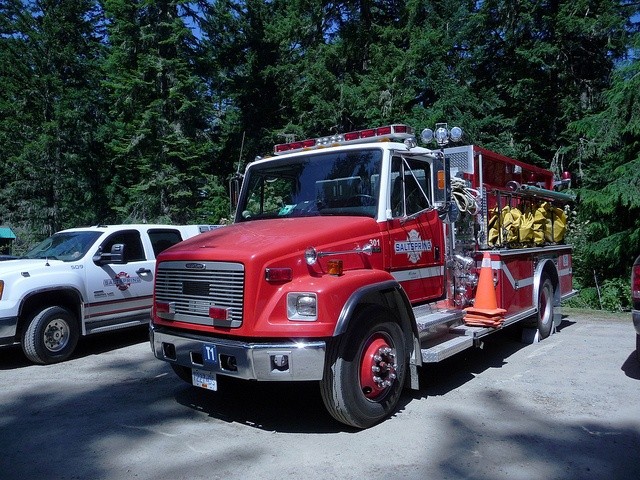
xmin=1 ymin=225 xmax=221 ymax=365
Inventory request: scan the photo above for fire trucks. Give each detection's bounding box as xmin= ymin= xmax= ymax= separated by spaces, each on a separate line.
xmin=148 ymin=121 xmax=577 ymax=428
